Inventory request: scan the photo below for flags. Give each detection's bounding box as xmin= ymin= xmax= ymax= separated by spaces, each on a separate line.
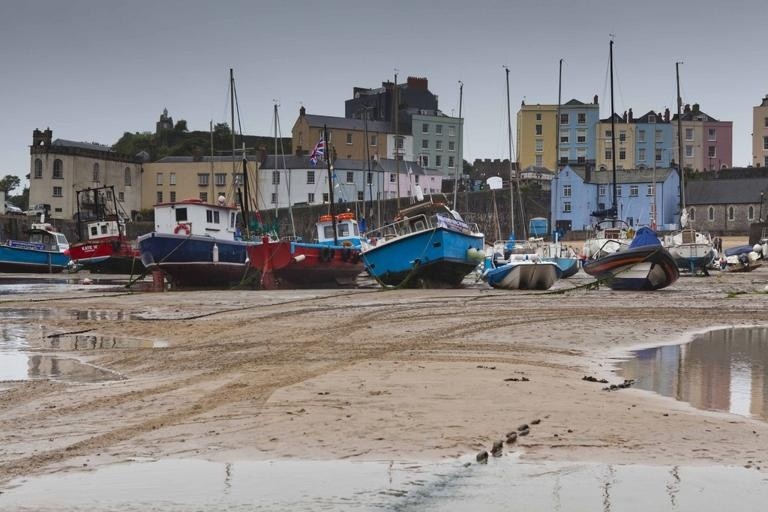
xmin=309 ymin=132 xmax=328 ymax=169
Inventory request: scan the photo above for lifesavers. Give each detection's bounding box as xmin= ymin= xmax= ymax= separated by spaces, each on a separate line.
xmin=174 ymin=224 xmax=190 ymax=235
